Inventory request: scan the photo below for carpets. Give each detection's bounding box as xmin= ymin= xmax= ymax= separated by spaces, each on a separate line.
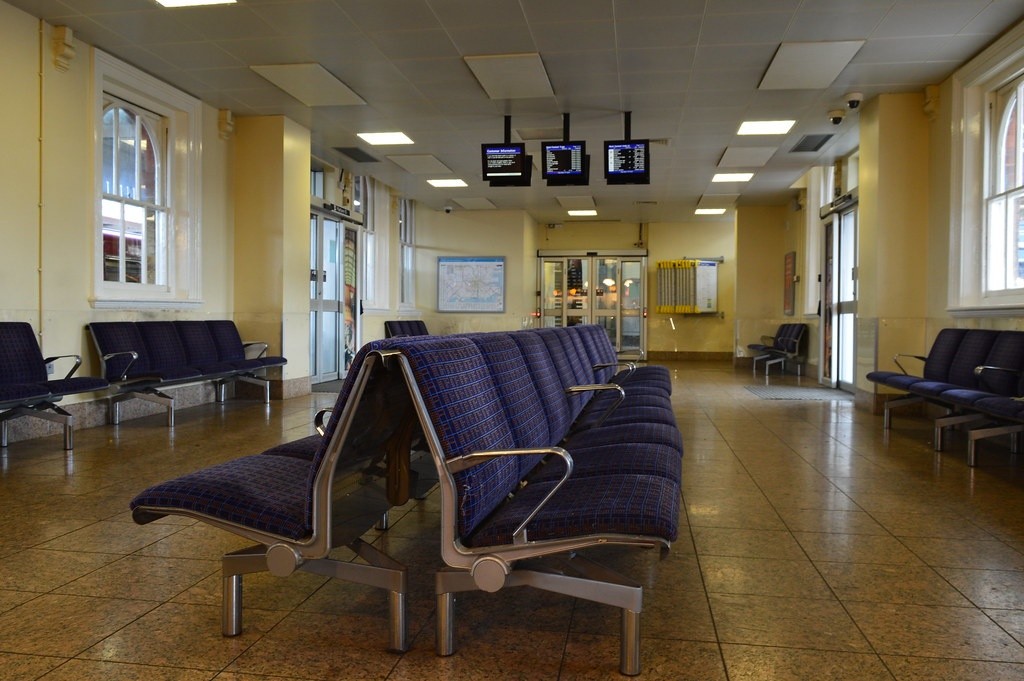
xmin=743 ymin=385 xmax=856 ymax=399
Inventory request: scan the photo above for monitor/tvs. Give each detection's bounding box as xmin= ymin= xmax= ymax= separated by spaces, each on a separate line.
xmin=481 ymin=143 xmax=533 ymax=186
xmin=540 ymin=140 xmax=591 ymax=186
xmin=604 ymin=139 xmax=650 ymax=185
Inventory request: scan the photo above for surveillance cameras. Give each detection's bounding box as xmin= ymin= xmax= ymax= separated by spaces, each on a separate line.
xmin=828 ymin=111 xmax=845 ymax=126
xmin=444 ymin=207 xmax=452 ymax=214
xmin=845 ymin=93 xmax=863 ymax=109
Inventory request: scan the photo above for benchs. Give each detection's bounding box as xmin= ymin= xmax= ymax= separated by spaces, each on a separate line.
xmin=1 ymin=320 xmax=683 ymax=677
xmin=747 ymin=323 xmax=811 ymax=385
xmin=867 ymin=327 xmax=1024 ymax=466
